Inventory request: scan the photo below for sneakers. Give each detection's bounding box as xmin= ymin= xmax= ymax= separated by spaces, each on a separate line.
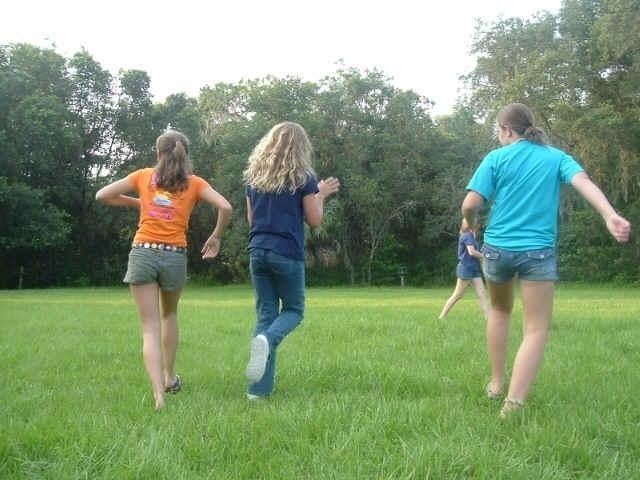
xmin=246 ymin=335 xmax=269 ymax=384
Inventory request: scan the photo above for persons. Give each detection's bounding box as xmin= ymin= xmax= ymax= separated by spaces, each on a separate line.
xmin=241 ymin=121 xmax=341 ymax=400
xmin=438 ymin=216 xmax=489 ymax=321
xmin=460 ymin=103 xmax=631 ymax=417
xmin=95 ymin=130 xmax=233 ymax=411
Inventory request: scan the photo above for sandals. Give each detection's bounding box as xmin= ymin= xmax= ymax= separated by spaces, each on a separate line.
xmin=165 ymin=375 xmax=181 ymax=394
